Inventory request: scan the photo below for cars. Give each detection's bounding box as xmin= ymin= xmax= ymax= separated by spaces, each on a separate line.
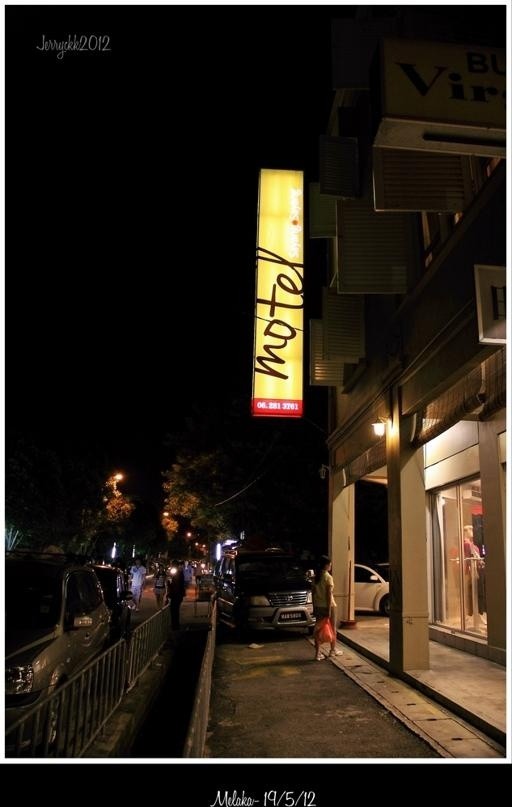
xmin=210 ymin=545 xmax=389 ymax=636
xmin=6 ymin=550 xmax=143 ymax=751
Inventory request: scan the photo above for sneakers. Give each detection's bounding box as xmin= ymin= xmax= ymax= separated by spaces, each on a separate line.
xmin=132 ymin=606 xmax=139 ymax=612
xmin=314 ymin=652 xmax=326 ymax=662
xmin=328 ymin=648 xmax=344 ymax=657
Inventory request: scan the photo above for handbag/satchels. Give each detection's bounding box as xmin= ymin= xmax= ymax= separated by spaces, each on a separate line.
xmin=313 ymin=619 xmax=336 ymax=646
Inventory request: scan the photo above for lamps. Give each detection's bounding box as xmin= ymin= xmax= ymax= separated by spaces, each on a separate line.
xmin=371 ymin=416 xmax=387 ymax=439
xmin=318 ymin=462 xmax=328 ymax=479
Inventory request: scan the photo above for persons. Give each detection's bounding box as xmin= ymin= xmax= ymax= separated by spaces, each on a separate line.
xmin=166 ymin=559 xmax=185 ymax=627
xmin=152 ymin=563 xmax=168 ymax=610
xmin=129 ymin=558 xmax=147 ymax=613
xmin=310 ymin=554 xmax=343 ymax=660
xmin=462 ymin=523 xmax=487 ymax=633
